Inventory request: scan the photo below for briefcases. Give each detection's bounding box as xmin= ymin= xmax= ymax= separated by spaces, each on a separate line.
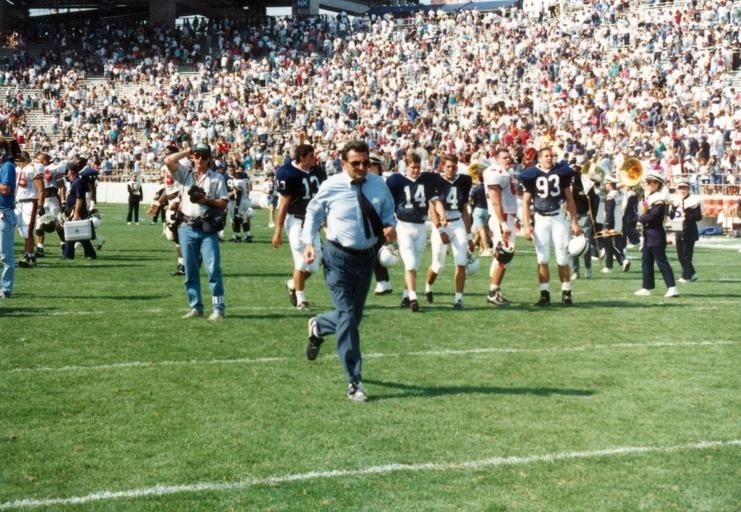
xmin=63 ymin=220 xmax=91 ymax=241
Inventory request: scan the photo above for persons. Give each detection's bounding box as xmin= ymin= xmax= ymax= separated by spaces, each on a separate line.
xmin=152 ymin=147 xmax=254 ymax=242
xmin=15 ymin=148 xmax=100 ymax=268
xmin=264 ymin=150 xmax=702 ymax=310
xmin=165 ymin=144 xmax=229 ymax=320
xmin=128 ymin=172 xmax=143 ymax=225
xmin=300 ymin=139 xmax=398 ymax=402
xmin=0 ymin=0 xmax=740 ymax=195
xmin=1 ymin=137 xmax=17 ymax=298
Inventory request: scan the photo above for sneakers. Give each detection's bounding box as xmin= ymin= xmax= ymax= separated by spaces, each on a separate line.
xmin=562 ymin=290 xmax=571 ymax=305
xmin=374 ymin=281 xmax=509 ymax=312
xmin=634 ymin=289 xmax=650 ymax=295
xmin=306 ymin=318 xmax=323 ymax=360
xmin=677 ymin=273 xmax=697 ymax=283
xmin=17 ymin=247 xmax=43 ymax=266
xmin=663 ymin=287 xmax=679 ymax=297
xmin=569 ymin=259 xmax=630 ymax=280
xmin=347 ymin=381 xmax=368 ymax=402
xmin=180 ymin=308 xmax=202 ymax=319
xmin=534 ymin=290 xmax=549 ymax=306
xmin=207 ymin=308 xmax=224 ymax=320
xmin=285 ymin=278 xmax=309 ymax=311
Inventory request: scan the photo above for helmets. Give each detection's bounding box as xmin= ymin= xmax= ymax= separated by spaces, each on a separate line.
xmin=464 ymin=258 xmax=479 ymax=275
xmin=495 ymin=241 xmax=514 ymax=263
xmin=377 ymin=243 xmax=398 ymax=267
xmin=568 ymin=234 xmax=588 ymax=257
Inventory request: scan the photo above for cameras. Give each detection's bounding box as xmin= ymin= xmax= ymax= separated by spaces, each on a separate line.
xmin=188 ymin=185 xmax=206 ymax=204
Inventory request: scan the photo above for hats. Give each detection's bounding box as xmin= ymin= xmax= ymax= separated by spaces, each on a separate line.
xmin=191 ymin=144 xmax=210 ymax=153
xmin=676 ymin=178 xmax=691 ymax=189
xmin=590 ymin=175 xmax=619 ymax=183
xmin=645 ymin=172 xmax=665 ymax=184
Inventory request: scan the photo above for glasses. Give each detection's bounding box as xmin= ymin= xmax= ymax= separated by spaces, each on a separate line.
xmin=194 ymin=153 xmax=208 ymax=160
xmin=193 ymin=153 xmax=210 ymax=160
xmin=346 ymin=160 xmax=369 ymax=167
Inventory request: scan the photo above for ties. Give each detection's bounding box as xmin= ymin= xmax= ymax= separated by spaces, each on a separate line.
xmin=355 ymin=183 xmax=383 ymax=238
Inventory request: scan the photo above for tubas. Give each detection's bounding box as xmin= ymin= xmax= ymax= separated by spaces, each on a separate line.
xmin=619 ymin=158 xmax=649 ymax=213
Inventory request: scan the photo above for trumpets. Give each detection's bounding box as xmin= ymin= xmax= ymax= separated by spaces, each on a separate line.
xmin=594 ymin=230 xmax=622 ymax=239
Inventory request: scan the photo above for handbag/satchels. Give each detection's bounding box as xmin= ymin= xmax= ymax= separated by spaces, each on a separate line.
xmin=200 ymin=211 xmax=225 ymax=232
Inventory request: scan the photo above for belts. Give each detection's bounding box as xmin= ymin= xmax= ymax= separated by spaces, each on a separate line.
xmin=446 ymin=218 xmax=459 ymax=222
xmin=538 ymin=212 xmax=559 ymax=216
